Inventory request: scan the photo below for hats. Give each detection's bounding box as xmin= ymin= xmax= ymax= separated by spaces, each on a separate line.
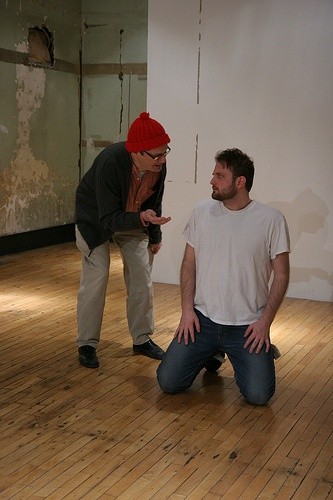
xmin=127 ymin=113 xmax=170 ymax=151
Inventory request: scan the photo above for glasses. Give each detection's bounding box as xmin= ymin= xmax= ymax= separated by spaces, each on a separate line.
xmin=143 ymin=144 xmax=171 ymax=160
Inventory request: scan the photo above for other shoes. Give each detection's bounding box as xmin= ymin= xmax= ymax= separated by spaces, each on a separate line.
xmin=271 ymin=344 xmax=281 ymax=359
xmin=204 ymin=351 xmax=225 ymax=371
xmin=133 ymin=339 xmax=164 ymax=359
xmin=79 ymin=346 xmax=99 ymax=368
